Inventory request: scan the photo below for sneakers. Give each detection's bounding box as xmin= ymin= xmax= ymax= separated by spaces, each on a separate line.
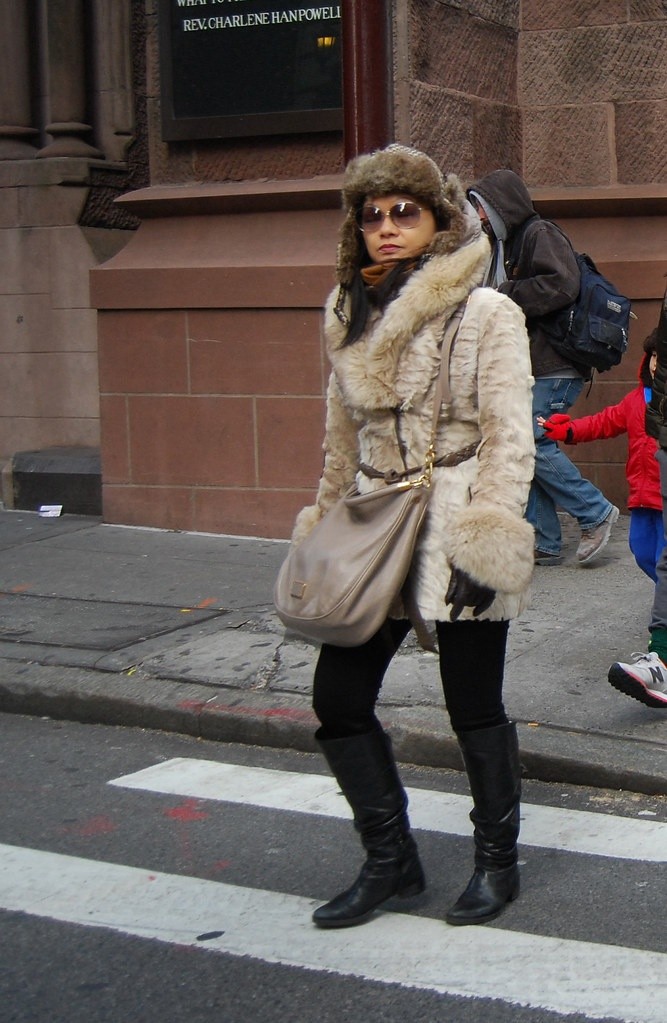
xmin=608 ymin=651 xmax=667 ymax=708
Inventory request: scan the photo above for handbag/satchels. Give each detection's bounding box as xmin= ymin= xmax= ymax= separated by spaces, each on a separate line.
xmin=540 ymin=219 xmax=638 ymax=372
xmin=274 ymin=479 xmax=433 ymax=652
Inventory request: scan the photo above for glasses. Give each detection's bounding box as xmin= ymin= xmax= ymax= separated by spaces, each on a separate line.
xmin=354 ymin=200 xmax=432 ymax=233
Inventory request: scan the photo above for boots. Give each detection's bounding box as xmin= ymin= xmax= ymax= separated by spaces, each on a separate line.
xmin=312 ymin=726 xmax=426 ymax=927
xmin=447 ymin=721 xmax=522 ymax=926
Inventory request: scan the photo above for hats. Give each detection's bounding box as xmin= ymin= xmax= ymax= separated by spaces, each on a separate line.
xmin=333 ymin=144 xmax=466 ymax=327
xmin=638 ymin=327 xmax=657 ymax=386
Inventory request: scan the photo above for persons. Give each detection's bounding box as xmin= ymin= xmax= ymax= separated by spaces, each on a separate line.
xmin=287 ymin=144 xmax=536 ymax=926
xmin=607 ymin=290 xmax=667 ymax=709
xmin=537 ymin=328 xmax=667 ymax=584
xmin=466 ymin=169 xmax=620 ymax=566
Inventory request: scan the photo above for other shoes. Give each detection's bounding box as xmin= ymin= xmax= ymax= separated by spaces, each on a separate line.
xmin=577 ymin=504 xmax=620 ymax=563
xmin=533 ymin=550 xmax=562 ymax=566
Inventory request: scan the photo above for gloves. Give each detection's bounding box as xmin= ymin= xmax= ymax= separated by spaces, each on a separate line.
xmin=445 ymin=566 xmax=495 ymax=621
